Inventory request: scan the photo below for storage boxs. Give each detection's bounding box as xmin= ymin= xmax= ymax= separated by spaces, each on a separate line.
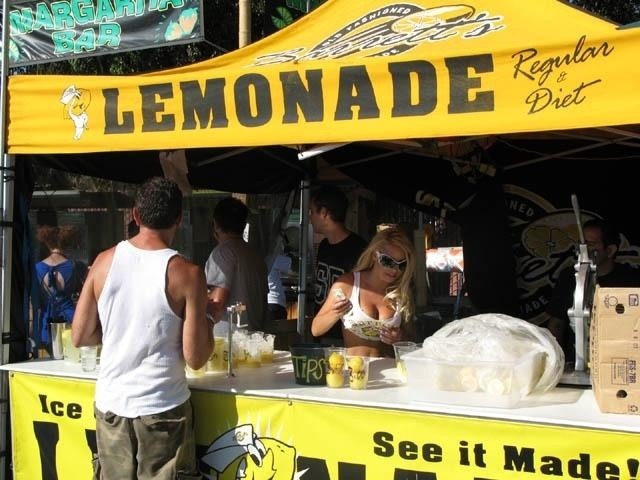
xmin=400 ymin=348 xmax=546 ymax=410
xmin=588 ymin=283 xmax=640 ymax=416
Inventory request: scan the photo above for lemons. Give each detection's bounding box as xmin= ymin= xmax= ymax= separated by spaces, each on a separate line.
xmin=328 ymin=352 xmax=344 ymax=373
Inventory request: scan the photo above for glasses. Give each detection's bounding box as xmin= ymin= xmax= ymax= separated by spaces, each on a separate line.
xmin=376 ymin=250 xmax=407 ymax=272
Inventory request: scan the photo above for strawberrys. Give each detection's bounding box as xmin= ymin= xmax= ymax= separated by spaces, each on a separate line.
xmin=179 ymin=6 xmax=199 ymax=35
xmin=165 ymin=22 xmax=185 ymax=42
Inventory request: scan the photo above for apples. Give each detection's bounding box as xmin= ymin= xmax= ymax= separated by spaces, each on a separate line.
xmin=348 ymin=356 xmax=364 ymax=373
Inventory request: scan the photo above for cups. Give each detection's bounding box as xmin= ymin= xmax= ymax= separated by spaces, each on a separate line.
xmin=78 ymin=344 xmax=98 ymax=374
xmin=321 ymin=347 xmax=348 ymax=389
xmin=223 ymin=328 xmax=276 ymax=371
xmin=50 ymin=322 xmax=80 ymax=365
xmin=392 ymin=341 xmax=417 ymax=384
xmin=345 ymin=352 xmax=371 ymax=391
xmin=185 ymin=335 xmax=225 ymax=378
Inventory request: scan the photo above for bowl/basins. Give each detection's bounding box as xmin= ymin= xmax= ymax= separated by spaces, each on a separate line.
xmin=287 ymin=346 xmax=336 ymax=383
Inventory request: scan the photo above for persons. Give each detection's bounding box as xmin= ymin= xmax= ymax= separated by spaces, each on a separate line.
xmin=30 ymin=224 xmax=89 ymax=358
xmin=69 ymin=175 xmax=224 ymax=480
xmin=201 ymin=196 xmax=260 ymax=331
xmin=309 ymin=226 xmax=418 ymax=360
xmin=548 ymin=217 xmax=639 ymax=365
xmin=458 ymin=272 xmax=493 ymax=314
xmin=305 ymin=184 xmax=372 ymax=346
xmin=263 ymin=250 xmax=293 ymax=318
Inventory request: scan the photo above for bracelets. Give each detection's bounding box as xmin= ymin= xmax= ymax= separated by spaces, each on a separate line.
xmin=205 ymin=313 xmax=216 ymax=325
